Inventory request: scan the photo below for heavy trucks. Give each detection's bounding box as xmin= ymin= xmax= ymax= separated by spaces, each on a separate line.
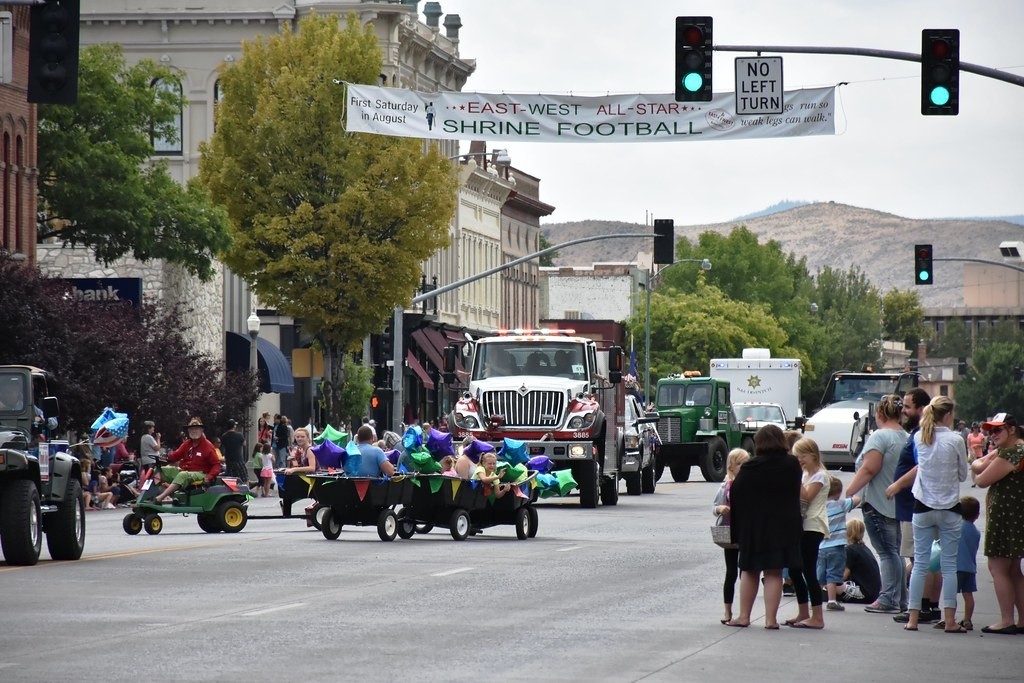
xmin=650 ymin=347 xmax=759 ymax=482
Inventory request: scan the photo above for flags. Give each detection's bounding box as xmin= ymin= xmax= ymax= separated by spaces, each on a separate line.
xmin=628 ymin=352 xmax=641 ymax=382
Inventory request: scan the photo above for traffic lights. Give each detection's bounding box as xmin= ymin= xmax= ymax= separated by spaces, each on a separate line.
xmin=372 ymin=394 xmax=379 ymax=419
xmin=915 ymin=244 xmax=934 ymax=286
xmin=675 ymin=15 xmax=714 ymax=102
xmin=920 ymin=28 xmax=960 ymax=116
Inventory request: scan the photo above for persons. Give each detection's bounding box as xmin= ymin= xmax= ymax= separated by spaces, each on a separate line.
xmin=712 ymin=387 xmax=1024 ymax=635
xmin=0 ymin=376 xmax=259 ymax=510
xmin=252 ymin=412 xmax=510 ymax=499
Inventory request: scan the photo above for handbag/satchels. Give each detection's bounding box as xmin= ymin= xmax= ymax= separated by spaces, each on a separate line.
xmin=968 ymin=448 xmax=978 ymax=463
xmin=711 ymin=511 xmax=731 ymax=543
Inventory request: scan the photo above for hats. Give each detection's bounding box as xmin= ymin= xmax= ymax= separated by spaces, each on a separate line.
xmin=958 ymin=420 xmax=966 ymax=425
xmin=227 ymin=419 xmax=238 ymax=426
xmin=972 ymin=421 xmax=979 ymax=428
xmin=182 ymin=417 xmax=209 ymax=430
xmin=981 ymin=412 xmax=1018 ymax=431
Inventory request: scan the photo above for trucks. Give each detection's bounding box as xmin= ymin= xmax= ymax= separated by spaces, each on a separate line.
xmin=709 ymin=347 xmax=802 ymax=437
xmin=443 ymin=329 xmax=625 ymax=510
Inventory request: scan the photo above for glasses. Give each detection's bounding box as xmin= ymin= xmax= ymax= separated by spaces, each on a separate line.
xmin=987 ymin=426 xmax=1004 ymax=435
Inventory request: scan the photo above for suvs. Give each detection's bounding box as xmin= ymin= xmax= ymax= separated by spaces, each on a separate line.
xmin=0 ymin=364 xmax=86 ymax=568
xmin=621 ymin=394 xmax=663 ymax=497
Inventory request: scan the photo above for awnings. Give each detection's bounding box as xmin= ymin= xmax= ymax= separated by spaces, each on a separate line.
xmin=372 ymin=318 xmax=468 ymax=391
xmin=227 ymin=330 xmax=295 ymax=395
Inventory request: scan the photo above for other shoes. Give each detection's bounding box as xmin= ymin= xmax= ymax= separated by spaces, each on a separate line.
xmin=103 ymin=503 xmax=117 ymax=509
xmin=86 ymin=507 xmax=97 ymax=511
xmin=893 ymin=613 xmax=931 ymax=624
xmin=863 ymin=601 xmax=900 ymax=613
xmin=958 ymin=620 xmax=973 ymax=631
xmin=981 ymin=623 xmax=1023 ymax=635
xmin=152 ymin=498 xmax=162 ymax=506
xmin=934 ymin=621 xmax=945 ymax=629
xmin=827 ymin=601 xmax=845 ymax=611
xmin=721 ymin=616 xmax=731 ymax=624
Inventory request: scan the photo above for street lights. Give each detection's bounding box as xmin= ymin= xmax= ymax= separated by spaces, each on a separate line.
xmin=246 ymin=307 xmax=261 ymax=468
xmin=647 ymin=257 xmax=712 ymax=410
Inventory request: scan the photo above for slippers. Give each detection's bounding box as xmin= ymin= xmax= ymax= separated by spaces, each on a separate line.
xmin=789 ymin=620 xmax=823 ymax=629
xmin=944 ymin=625 xmax=967 ymax=633
xmin=765 ymin=622 xmax=779 ymax=629
xmin=904 ymin=623 xmax=918 ymax=631
xmin=725 ymin=618 xmax=749 ymax=627
xmin=780 ymin=617 xmax=797 ymax=626
